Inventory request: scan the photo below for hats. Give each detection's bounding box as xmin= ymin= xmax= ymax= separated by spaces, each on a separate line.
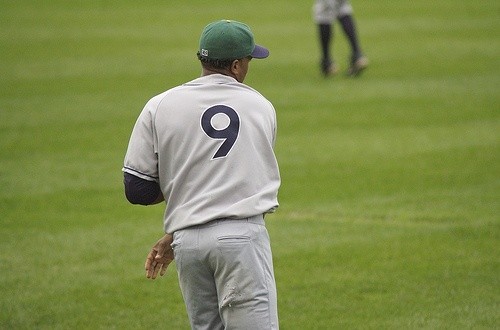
xmin=199 ymin=19 xmax=270 ymax=61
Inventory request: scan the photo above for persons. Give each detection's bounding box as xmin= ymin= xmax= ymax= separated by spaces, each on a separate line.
xmin=312 ymin=0 xmax=367 ymax=79
xmin=120 ymin=19 xmax=282 ymax=330
